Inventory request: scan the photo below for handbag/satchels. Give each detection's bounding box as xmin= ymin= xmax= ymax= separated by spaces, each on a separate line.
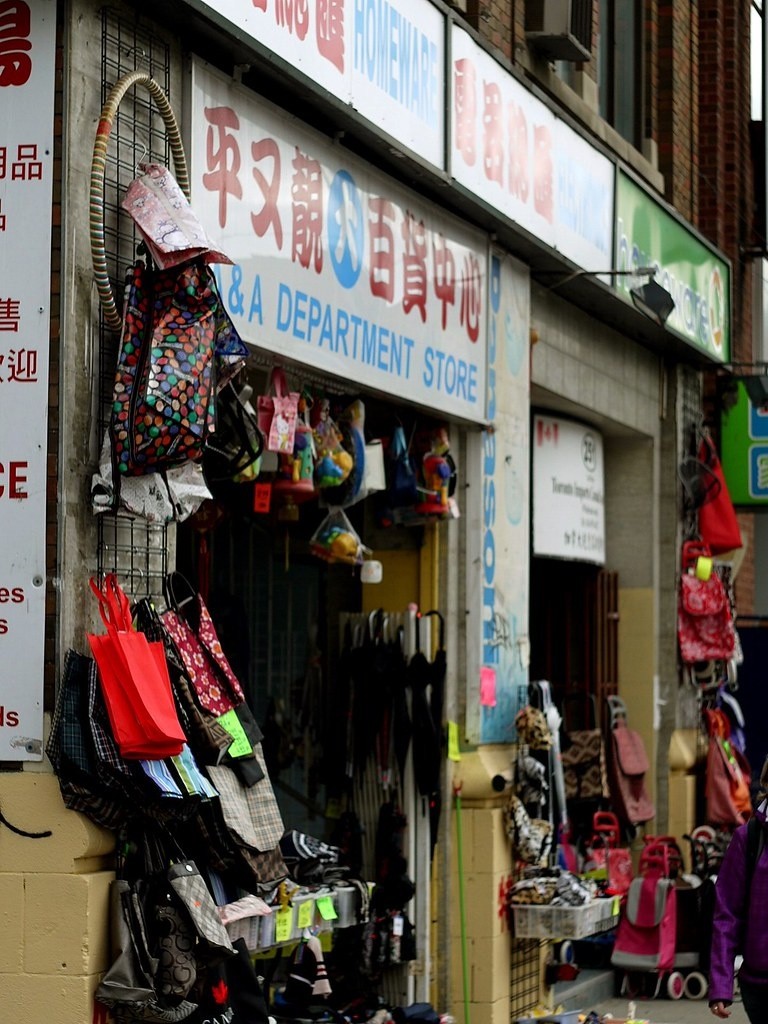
xmin=47 ymin=574 xmax=290 ymax=1024
xmin=676 ymin=435 xmax=755 ymax=828
xmin=94 ymin=162 xmax=302 ymax=529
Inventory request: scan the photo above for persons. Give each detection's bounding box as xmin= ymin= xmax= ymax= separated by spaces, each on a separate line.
xmin=708 ymin=761 xmax=768 ymax=1024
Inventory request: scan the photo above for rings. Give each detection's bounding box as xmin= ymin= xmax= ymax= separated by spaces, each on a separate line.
xmin=715 ymin=1006 xmax=718 ymax=1010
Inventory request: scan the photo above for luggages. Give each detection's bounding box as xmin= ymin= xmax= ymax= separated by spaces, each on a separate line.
xmin=606 ymin=695 xmax=655 ymax=842
xmin=560 ymin=811 xmax=736 ymax=1001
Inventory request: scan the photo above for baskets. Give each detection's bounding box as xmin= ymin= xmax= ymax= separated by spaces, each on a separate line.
xmin=511 ymin=896 xmax=619 ymax=939
